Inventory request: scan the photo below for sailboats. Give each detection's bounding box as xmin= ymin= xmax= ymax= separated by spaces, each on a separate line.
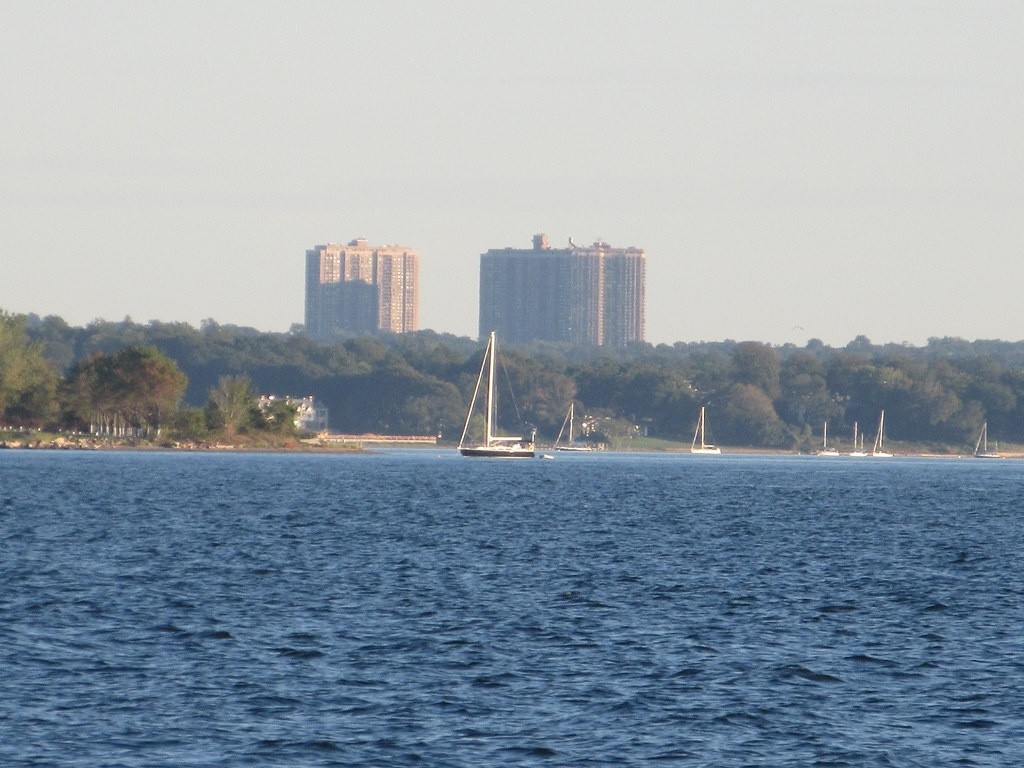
xmin=848 ymin=421 xmax=867 ymax=457
xmin=816 ymin=421 xmax=839 ymax=457
xmin=870 ymin=410 xmax=893 ymax=457
xmin=457 ymin=331 xmax=538 ymax=457
xmin=974 ymin=421 xmax=1000 ymax=458
xmin=690 ymin=406 xmax=721 ymax=453
xmin=552 ymin=403 xmax=592 ymax=451
